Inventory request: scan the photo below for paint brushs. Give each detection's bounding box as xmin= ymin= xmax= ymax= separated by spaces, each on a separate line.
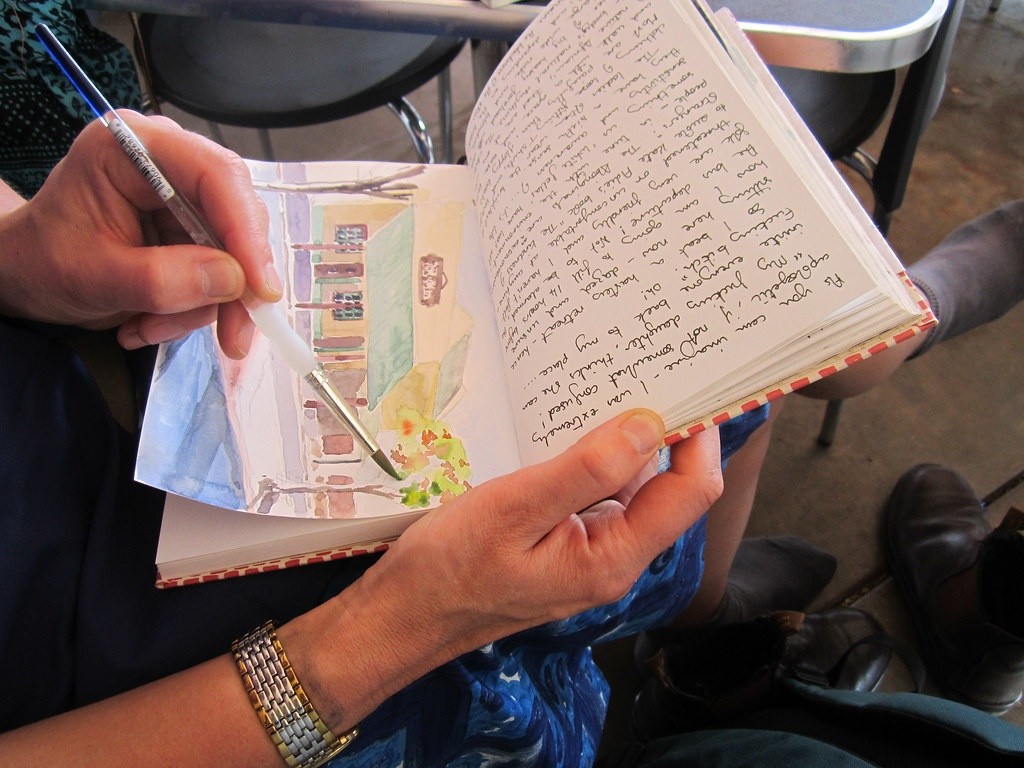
xmin=31 ymin=21 xmax=404 ymax=481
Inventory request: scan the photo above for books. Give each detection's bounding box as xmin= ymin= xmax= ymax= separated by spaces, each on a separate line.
xmin=133 ymin=1 xmax=939 ymax=592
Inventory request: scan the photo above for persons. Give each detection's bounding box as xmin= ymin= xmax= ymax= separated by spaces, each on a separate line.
xmin=0 ymin=104 xmax=894 ymax=767
xmin=794 ymin=200 xmax=1024 ymax=404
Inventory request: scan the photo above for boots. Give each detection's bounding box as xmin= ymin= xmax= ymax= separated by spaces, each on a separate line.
xmin=887 ymin=463 xmax=1023 ymax=716
xmin=627 ymin=609 xmax=892 ymax=748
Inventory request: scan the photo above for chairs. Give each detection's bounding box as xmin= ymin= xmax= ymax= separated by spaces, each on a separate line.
xmin=488 ymin=0 xmax=971 ymax=446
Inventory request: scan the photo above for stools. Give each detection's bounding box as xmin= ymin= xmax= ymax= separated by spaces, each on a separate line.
xmin=134 ymin=13 xmax=468 ymax=175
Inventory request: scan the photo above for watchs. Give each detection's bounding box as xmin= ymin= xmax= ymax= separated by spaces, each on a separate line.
xmin=230 ymin=619 xmax=361 ymax=768
